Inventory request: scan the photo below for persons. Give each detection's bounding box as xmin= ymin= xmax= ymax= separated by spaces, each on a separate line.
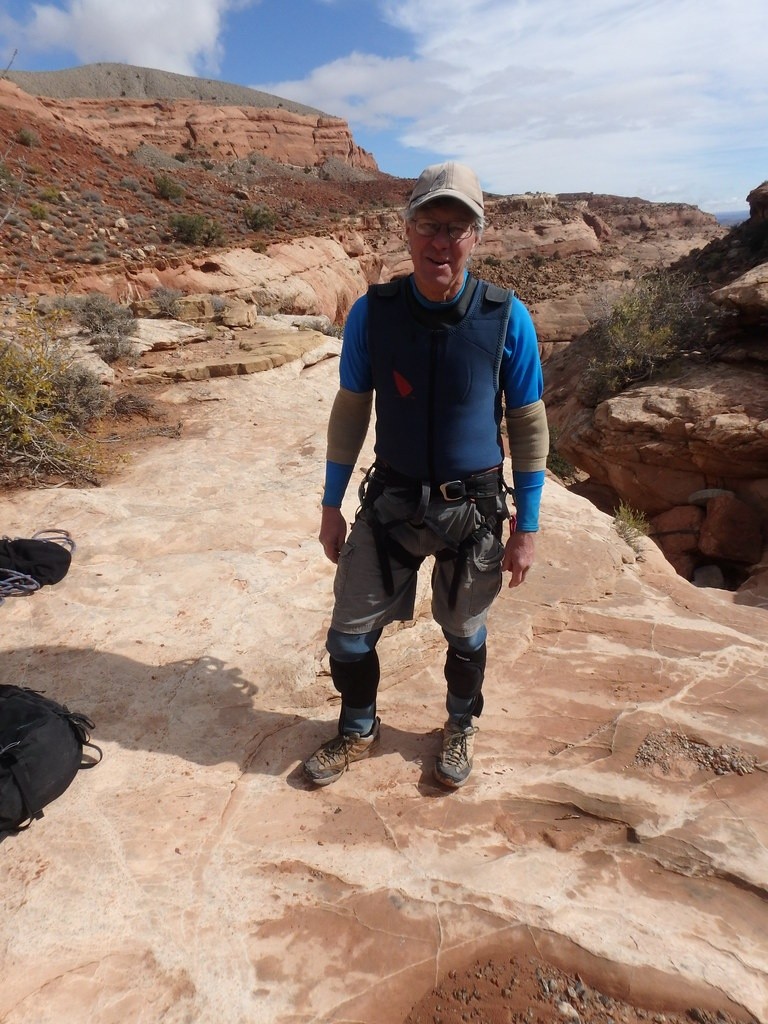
xmin=300 ymin=161 xmax=553 ymax=790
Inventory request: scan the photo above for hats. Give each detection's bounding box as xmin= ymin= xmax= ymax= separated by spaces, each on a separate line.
xmin=409 ymin=162 xmax=484 ymax=219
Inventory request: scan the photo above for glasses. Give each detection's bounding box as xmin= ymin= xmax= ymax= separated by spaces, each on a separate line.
xmin=409 ymin=217 xmax=477 ymax=239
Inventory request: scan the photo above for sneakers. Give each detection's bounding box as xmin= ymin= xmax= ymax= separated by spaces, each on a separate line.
xmin=433 ymin=721 xmax=479 ymax=788
xmin=304 ymin=716 xmax=381 ymax=785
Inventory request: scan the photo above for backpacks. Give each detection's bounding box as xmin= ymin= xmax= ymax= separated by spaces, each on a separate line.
xmin=1 ymin=683 xmax=101 ymax=832
